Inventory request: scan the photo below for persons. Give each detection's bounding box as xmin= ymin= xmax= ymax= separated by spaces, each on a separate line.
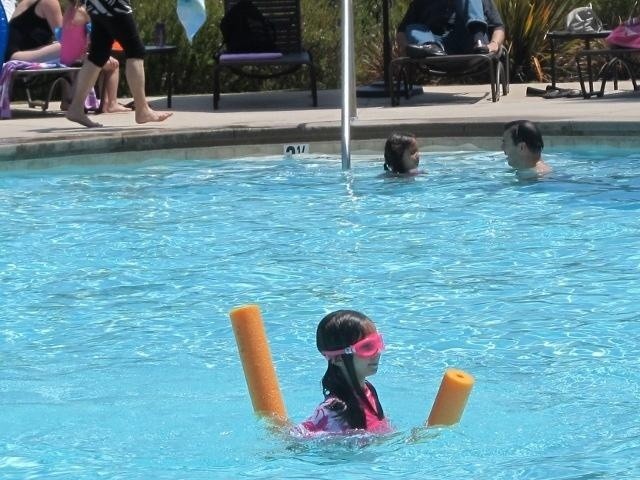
xmin=500 ymin=119 xmax=552 ymax=184
xmin=380 ymin=134 xmax=427 ymax=178
xmin=63 ymin=1 xmax=174 ymax=129
xmin=60 ymin=0 xmax=133 ymax=113
xmin=2 ymin=0 xmax=64 ymax=65
xmin=288 ymin=309 xmax=393 ymax=439
xmin=396 ymin=0 xmax=508 ymax=59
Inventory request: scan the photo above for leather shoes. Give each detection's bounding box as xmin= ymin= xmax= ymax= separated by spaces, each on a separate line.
xmin=472 ymin=40 xmax=490 ymax=55
xmin=406 ymin=42 xmax=448 ymax=58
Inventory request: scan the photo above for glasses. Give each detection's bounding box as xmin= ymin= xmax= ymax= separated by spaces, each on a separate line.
xmin=323 ymin=334 xmax=386 ymax=357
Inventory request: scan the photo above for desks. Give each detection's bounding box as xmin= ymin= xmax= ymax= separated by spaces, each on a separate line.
xmin=547 ymin=31 xmax=619 ymax=90
xmin=110 ymin=46 xmax=176 ymax=108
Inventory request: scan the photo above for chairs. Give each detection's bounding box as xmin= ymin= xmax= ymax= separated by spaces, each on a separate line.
xmin=575 ymin=0 xmax=640 ymax=99
xmin=388 ymin=45 xmax=510 ymax=106
xmin=212 ymin=1 xmax=318 ymax=109
xmin=0 ymin=0 xmax=106 ymax=115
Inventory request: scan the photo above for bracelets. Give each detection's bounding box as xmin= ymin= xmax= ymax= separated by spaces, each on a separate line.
xmin=491 ymin=40 xmax=500 ymax=54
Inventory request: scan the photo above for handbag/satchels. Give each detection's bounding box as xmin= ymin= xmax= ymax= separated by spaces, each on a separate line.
xmin=609 ymin=0 xmax=640 ymax=52
xmin=564 ymin=1 xmax=603 ymax=33
xmin=60 ymin=4 xmax=92 ymax=65
